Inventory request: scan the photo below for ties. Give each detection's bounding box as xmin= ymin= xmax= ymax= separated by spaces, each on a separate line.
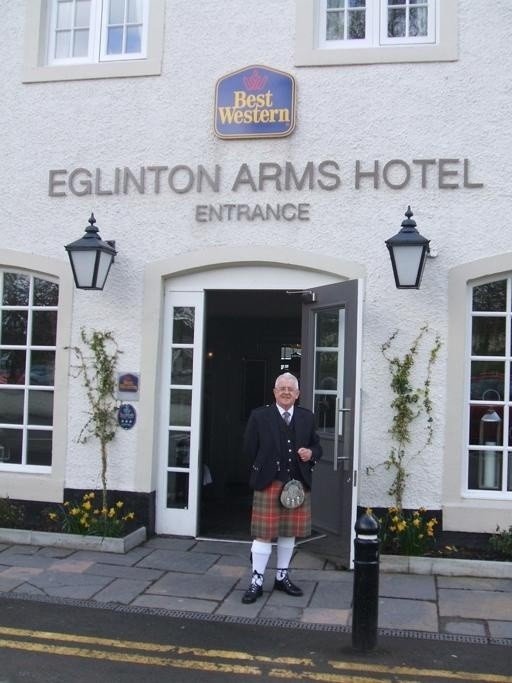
xmin=283 ymin=412 xmax=290 ymax=426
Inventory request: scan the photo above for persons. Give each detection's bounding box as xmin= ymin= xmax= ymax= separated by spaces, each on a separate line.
xmin=241 ymin=372 xmax=323 ymax=604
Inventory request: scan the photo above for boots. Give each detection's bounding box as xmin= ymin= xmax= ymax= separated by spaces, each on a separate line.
xmin=274 ymin=572 xmax=303 ymax=597
xmin=242 ymin=578 xmax=263 ymax=603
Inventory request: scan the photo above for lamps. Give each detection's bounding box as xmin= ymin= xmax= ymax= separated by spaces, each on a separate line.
xmin=382 ymin=208 xmax=436 ymax=290
xmin=62 ymin=212 xmax=118 ymax=291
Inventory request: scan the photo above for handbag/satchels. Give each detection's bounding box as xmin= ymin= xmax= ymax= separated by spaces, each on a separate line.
xmin=280 ymin=480 xmax=305 ymax=508
